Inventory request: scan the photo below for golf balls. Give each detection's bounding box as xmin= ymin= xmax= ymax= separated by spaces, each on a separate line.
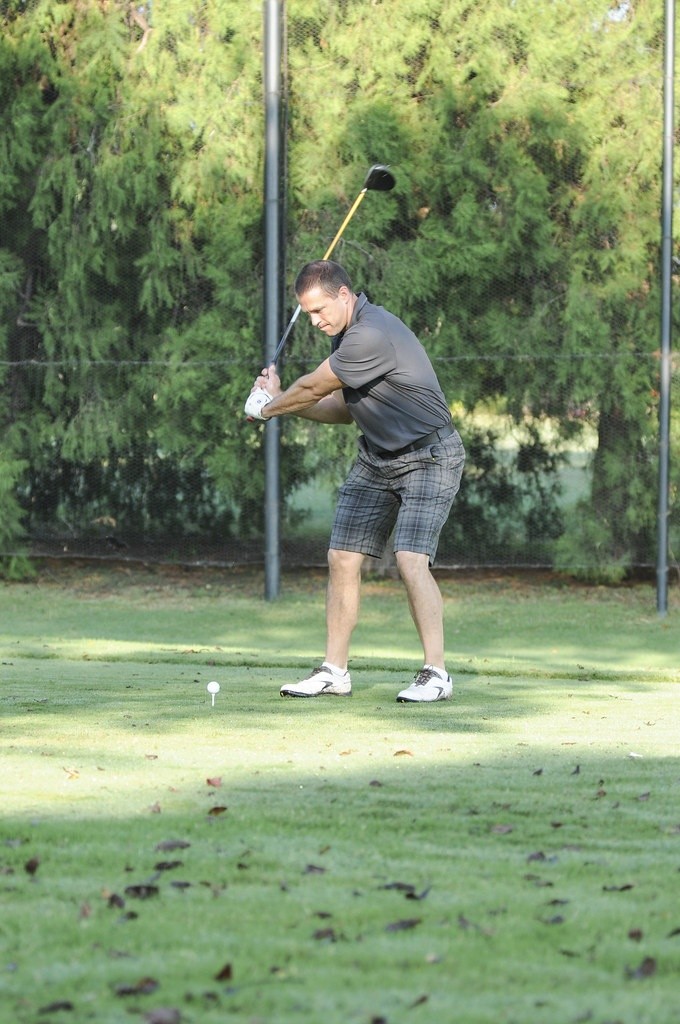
xmin=206 ymin=680 xmax=221 ymax=694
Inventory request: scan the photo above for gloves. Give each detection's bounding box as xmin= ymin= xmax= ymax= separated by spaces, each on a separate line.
xmin=244 ymin=388 xmax=273 ymax=421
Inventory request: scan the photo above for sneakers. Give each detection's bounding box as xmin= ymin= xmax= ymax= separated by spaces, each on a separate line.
xmin=396 ymin=664 xmax=453 ymax=703
xmin=279 ymin=666 xmax=352 ymax=697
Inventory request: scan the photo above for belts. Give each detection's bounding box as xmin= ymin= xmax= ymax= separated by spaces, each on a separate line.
xmin=379 ymin=423 xmax=454 ymax=460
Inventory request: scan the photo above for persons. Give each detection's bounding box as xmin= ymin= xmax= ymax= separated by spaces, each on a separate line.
xmin=242 ymin=259 xmax=466 ymax=702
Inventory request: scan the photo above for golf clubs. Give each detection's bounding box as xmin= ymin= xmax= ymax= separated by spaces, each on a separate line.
xmin=245 ymin=163 xmax=399 ymax=423
